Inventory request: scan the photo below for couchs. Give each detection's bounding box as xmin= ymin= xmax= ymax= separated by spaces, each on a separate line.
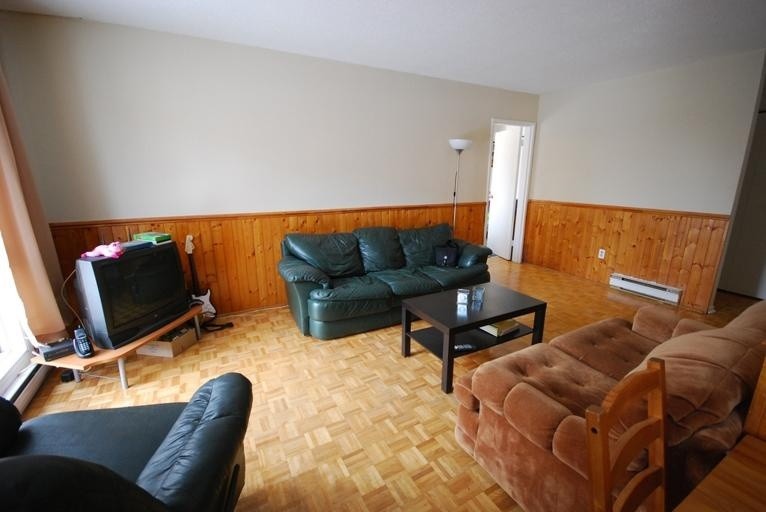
xmin=276 ymin=223 xmax=492 ymax=341
xmin=452 ymin=301 xmax=766 ymax=512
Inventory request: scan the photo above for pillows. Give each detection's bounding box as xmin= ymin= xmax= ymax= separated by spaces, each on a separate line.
xmin=433 ymin=246 xmax=457 ymax=269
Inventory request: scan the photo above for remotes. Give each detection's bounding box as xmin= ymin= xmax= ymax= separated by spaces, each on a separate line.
xmin=453 ymin=344 xmax=473 ymax=351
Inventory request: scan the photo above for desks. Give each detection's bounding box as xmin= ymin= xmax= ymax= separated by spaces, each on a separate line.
xmin=29 ymin=303 xmax=203 ymax=391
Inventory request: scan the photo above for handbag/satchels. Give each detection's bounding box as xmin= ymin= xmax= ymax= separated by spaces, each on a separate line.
xmin=434 ymin=239 xmax=462 ymax=267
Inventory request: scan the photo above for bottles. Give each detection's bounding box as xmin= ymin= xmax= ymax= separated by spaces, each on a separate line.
xmin=443 ymin=255 xmax=448 ymax=266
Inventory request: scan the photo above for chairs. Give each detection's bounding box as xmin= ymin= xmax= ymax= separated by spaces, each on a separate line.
xmin=585 ymin=357 xmax=668 ymax=512
xmin=0 ymin=371 xmax=253 ymax=512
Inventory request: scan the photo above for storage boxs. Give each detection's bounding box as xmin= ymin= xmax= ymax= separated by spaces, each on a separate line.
xmin=135 ymin=327 xmax=198 ymax=358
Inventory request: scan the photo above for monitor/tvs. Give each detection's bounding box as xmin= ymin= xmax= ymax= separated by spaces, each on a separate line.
xmin=74 ymin=240 xmax=189 ymax=350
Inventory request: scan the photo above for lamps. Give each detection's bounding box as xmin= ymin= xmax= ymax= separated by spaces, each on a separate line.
xmin=449 ymin=136 xmax=473 ymax=238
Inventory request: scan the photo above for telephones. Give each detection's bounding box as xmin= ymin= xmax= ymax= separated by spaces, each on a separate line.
xmin=73 ymin=324 xmax=93 ymax=358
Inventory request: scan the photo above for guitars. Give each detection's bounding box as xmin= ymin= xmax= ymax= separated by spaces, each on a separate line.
xmin=185 ymin=235 xmax=216 ymax=322
